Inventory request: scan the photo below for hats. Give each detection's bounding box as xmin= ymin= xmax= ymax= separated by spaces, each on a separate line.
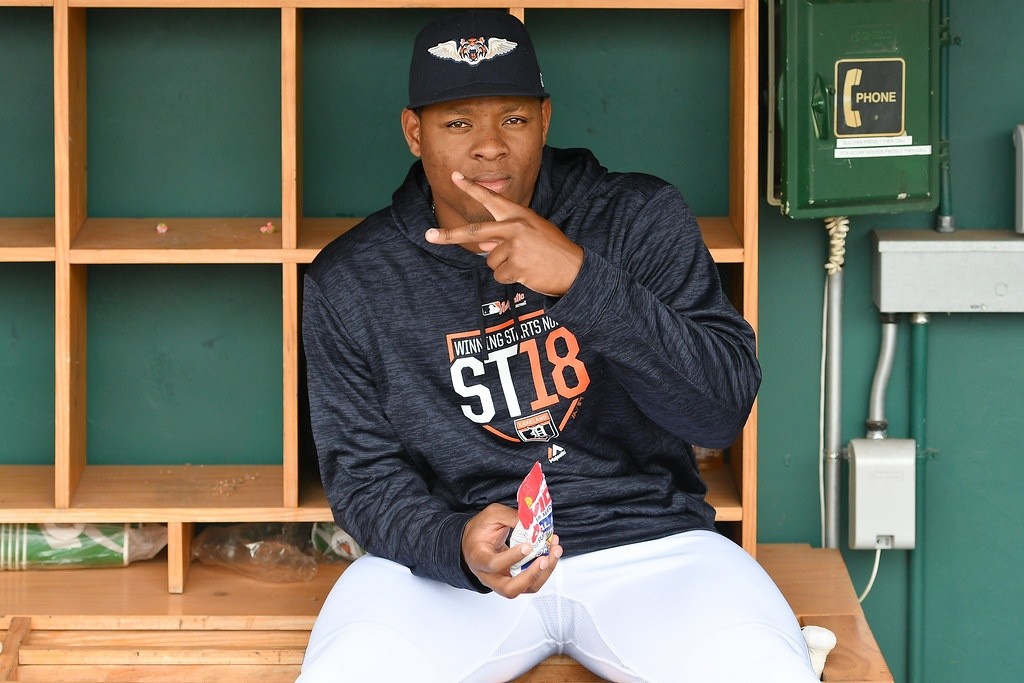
xmin=407 ymin=10 xmax=552 ymax=110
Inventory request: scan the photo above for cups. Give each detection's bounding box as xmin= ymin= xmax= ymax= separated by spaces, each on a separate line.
xmin=0 ymin=523 xmax=129 ymax=571
xmin=311 ymin=521 xmax=366 ymax=560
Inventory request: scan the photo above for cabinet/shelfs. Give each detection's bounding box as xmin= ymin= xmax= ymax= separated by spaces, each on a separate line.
xmin=0 ymin=1 xmax=758 ymax=595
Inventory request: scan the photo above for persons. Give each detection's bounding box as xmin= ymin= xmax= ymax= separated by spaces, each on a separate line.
xmin=294 ymin=5 xmax=841 ymax=683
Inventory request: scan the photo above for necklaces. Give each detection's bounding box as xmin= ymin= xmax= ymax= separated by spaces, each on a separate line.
xmin=431 ymin=198 xmax=435 ymax=217
